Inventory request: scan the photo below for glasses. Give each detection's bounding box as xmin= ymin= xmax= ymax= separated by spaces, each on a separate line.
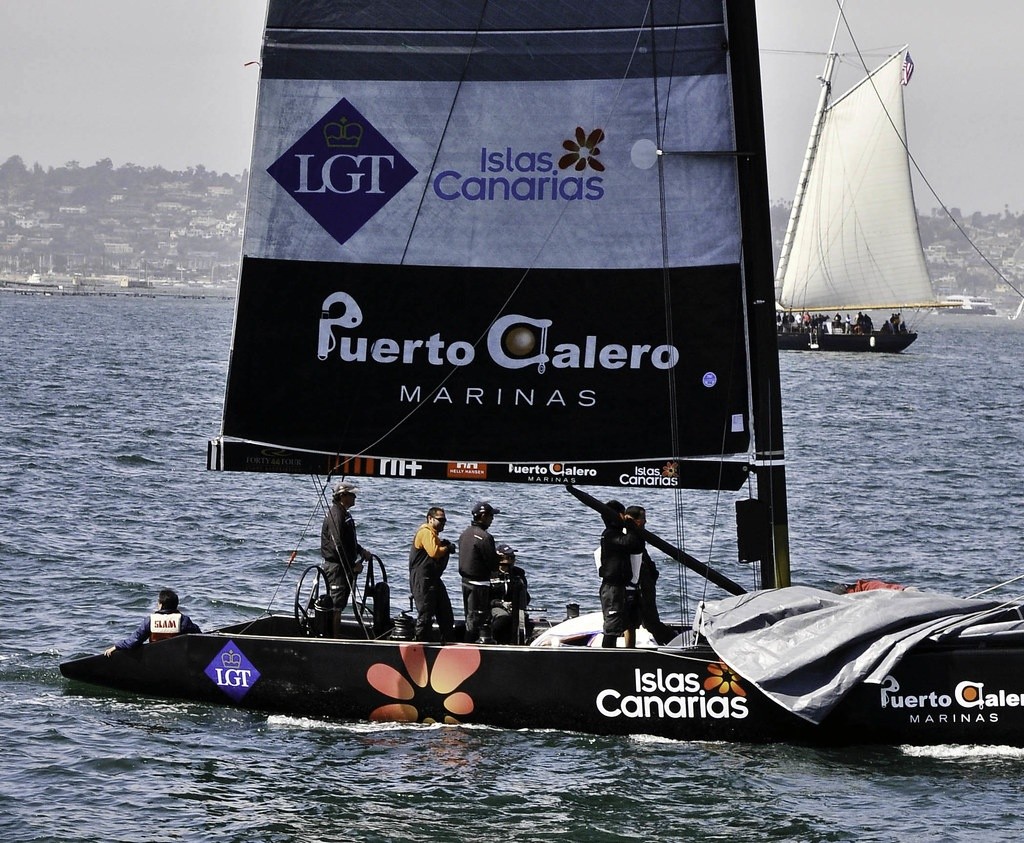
xmin=485 ymin=514 xmax=494 ymax=519
xmin=431 ymin=515 xmax=446 ymax=523
xmin=500 ymin=555 xmax=515 ymax=560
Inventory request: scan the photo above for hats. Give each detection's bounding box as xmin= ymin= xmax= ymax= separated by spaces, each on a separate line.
xmin=496 ymin=544 xmax=518 ymax=556
xmin=472 ymin=502 xmax=500 ymax=516
xmin=332 ymin=481 xmax=359 ymax=497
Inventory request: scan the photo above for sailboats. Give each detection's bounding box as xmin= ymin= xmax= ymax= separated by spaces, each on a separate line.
xmin=61 ymin=3 xmax=1024 ymax=755
xmin=772 ymin=0 xmax=967 ymax=353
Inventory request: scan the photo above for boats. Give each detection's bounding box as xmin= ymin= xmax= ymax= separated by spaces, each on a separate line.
xmin=935 ymin=293 xmax=996 ymax=316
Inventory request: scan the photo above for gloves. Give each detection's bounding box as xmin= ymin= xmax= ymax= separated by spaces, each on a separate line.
xmin=441 ymin=539 xmax=451 ymax=546
xmin=447 ymin=544 xmax=455 ymax=554
xmin=500 ymin=600 xmax=513 ymax=614
xmin=353 ymin=563 xmax=364 ymax=575
xmin=360 ymin=550 xmax=372 ymax=562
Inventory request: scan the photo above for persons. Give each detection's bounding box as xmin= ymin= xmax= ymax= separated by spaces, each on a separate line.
xmin=598 ymin=500 xmax=644 ymax=648
xmin=321 ymin=482 xmax=372 ymax=639
xmin=834 ymin=313 xmax=841 ymax=321
xmin=882 ymin=313 xmax=906 ymax=334
xmin=622 ymin=506 xmax=677 ymax=647
xmin=102 ymin=590 xmax=202 ymax=658
xmin=845 ymin=312 xmax=874 ymax=335
xmin=489 ymin=545 xmax=527 ymax=645
xmin=458 ymin=503 xmax=500 ymax=643
xmin=409 ymin=507 xmax=456 ymax=641
xmin=776 ymin=311 xmax=830 ymax=331
